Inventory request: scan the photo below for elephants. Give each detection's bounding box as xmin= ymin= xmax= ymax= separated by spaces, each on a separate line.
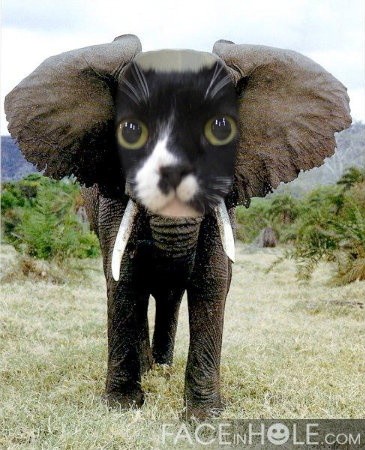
xmin=4 ymin=34 xmax=353 ymax=430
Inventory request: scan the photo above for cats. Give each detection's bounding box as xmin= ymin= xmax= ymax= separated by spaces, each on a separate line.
xmin=99 ymin=62 xmax=251 ymax=219
